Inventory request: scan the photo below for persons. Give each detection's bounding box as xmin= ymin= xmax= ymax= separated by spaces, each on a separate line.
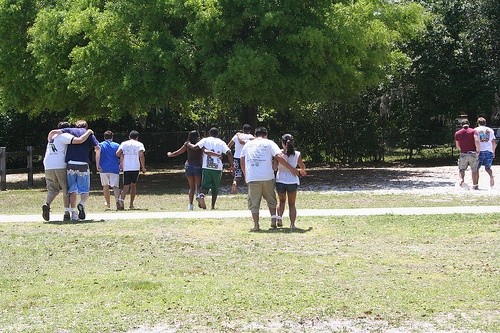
xmin=455 ymin=118 xmax=482 ymax=191
xmin=42 ymin=121 xmax=146 ymax=221
xmin=473 ymin=117 xmax=497 ymax=188
xmin=167 ymin=124 xmax=307 ymax=231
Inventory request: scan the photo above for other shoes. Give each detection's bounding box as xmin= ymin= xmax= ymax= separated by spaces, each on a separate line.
xmin=199 ymin=194 xmax=206 ymax=208
xmin=77 ymin=201 xmax=85 ymax=219
xmin=72 ymin=214 xmax=79 ymax=220
xmin=196 ymin=194 xmax=202 ymax=208
xmin=63 ymin=214 xmax=71 ymax=221
xmin=42 ymin=204 xmax=50 ymax=221
xmin=188 ymin=204 xmax=193 ymax=210
